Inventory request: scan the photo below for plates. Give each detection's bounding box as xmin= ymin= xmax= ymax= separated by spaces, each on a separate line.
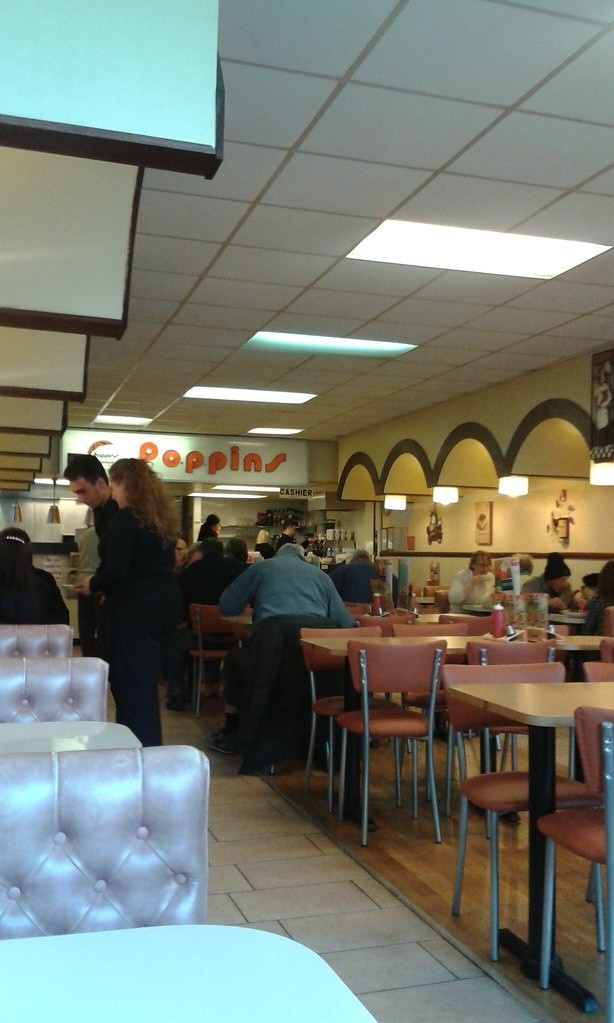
xmin=62 ymin=584 xmax=74 ymax=589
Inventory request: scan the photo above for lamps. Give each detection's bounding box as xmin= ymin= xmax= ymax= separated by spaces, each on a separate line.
xmin=498 ymin=475 xmax=528 ymax=497
xmin=384 ymin=495 xmax=407 ymax=511
xmin=48 ymin=477 xmax=61 ymax=523
xmin=432 ymin=485 xmax=459 ymax=506
xmin=590 ymin=459 xmax=614 ymax=487
xmin=10 ymin=491 xmax=22 ymax=523
xmin=85 ymin=507 xmax=95 ymax=527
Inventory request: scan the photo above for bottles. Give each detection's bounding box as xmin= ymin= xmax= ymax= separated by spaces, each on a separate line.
xmin=276 ymin=533 xmax=280 ymax=540
xmin=372 ymin=592 xmax=382 ymax=616
xmin=273 ymin=509 xmax=289 ymax=526
xmin=492 ymin=603 xmax=505 ymax=638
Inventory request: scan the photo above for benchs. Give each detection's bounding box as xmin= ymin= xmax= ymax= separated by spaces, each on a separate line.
xmin=0 ymin=744 xmax=211 ymax=941
xmin=0 ymin=624 xmax=110 ymax=722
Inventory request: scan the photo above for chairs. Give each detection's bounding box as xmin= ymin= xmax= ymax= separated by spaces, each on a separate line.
xmin=186 ymin=600 xmax=614 ymax=1023
xmin=433 ymin=589 xmax=449 ymax=613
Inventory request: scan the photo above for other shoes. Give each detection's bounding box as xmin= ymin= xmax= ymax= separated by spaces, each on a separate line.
xmin=205 ymin=731 xmax=237 ymax=754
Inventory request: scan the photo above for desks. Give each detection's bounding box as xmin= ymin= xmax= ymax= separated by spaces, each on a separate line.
xmin=410 ymin=613 xmax=478 ymax=625
xmin=417 ymin=597 xmax=433 ymax=605
xmin=462 ymin=603 xmax=494 ymax=614
xmin=447 ymin=687 xmax=613 ymax=1013
xmin=0 ymin=924 xmax=379 ymax=1023
xmin=0 ymin=722 xmax=144 ymax=756
xmin=547 ymin=613 xmax=588 ymax=624
xmin=300 ymin=635 xmax=614 ymax=834
xmin=222 ymin=616 xmax=254 ymax=628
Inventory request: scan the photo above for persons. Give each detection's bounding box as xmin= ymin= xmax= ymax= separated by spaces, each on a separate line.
xmin=329 ymin=549 xmax=399 ymax=603
xmin=448 ymin=550 xmax=496 ymax=615
xmin=516 ymin=553 xmax=535 ymax=585
xmin=276 ymin=520 xmax=314 ymax=556
xmin=0 ymin=527 xmax=70 ymax=625
xmin=63 ymin=454 xmax=180 ymax=747
xmin=255 ymin=530 xmax=276 ymax=560
xmin=522 ymin=551 xmax=587 ymax=614
xmin=565 ymin=561 xmax=614 ymax=683
xmin=166 ymin=537 xmax=249 ymax=711
xmin=198 ymin=514 xmax=222 ymax=542
xmin=204 ymin=544 xmax=358 ymax=755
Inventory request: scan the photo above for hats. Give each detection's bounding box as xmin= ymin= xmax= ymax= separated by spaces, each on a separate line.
xmin=543 ymin=553 xmax=571 ymax=580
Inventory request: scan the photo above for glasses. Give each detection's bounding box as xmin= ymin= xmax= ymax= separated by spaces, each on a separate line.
xmin=475 ymin=562 xmax=491 ymax=569
